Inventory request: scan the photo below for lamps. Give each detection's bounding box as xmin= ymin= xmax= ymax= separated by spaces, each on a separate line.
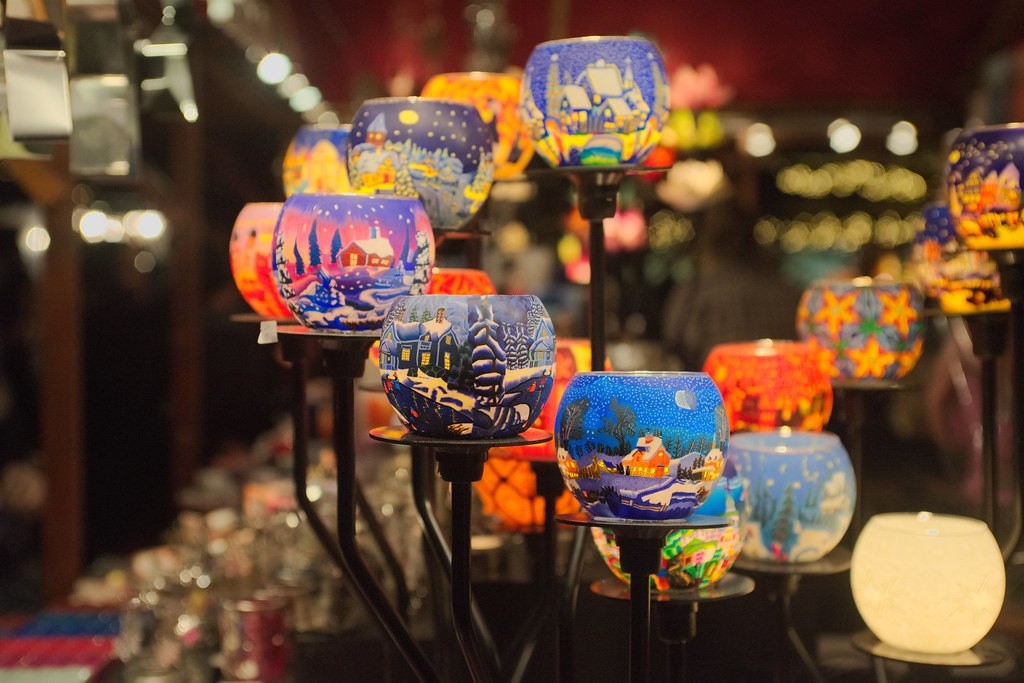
xmin=0 ymin=19 xmax=202 ymax=180
xmin=224 ymin=31 xmax=1024 ymax=683
